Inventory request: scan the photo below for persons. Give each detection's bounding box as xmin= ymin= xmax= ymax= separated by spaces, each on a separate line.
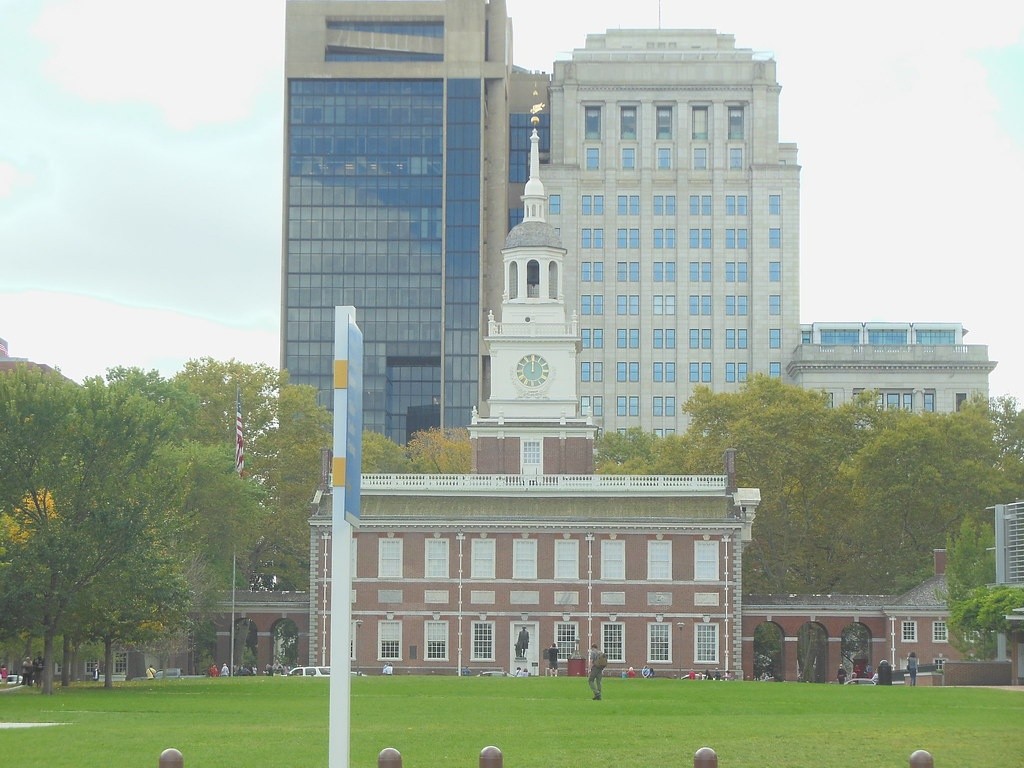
xmin=745 ymin=672 xmax=778 ymax=682
xmin=627 ymin=666 xmax=636 ymax=678
xmin=688 ymin=669 xmax=696 ymax=680
xmin=233 ymin=663 xmax=252 ymax=676
xmin=382 ymin=662 xmax=394 ymax=676
xmin=515 ymin=667 xmax=529 ymax=678
xmin=32 ymin=651 xmax=45 ymax=688
xmin=20 ymin=657 xmax=33 ymax=686
xmin=837 ymin=663 xmax=847 ymax=685
xmin=221 ymin=662 xmax=229 ymax=677
xmin=146 ymin=664 xmax=157 ymax=680
xmin=210 ymin=664 xmax=219 ymax=677
xmin=907 ymin=652 xmax=917 ymax=686
xmin=705 ymin=669 xmax=713 ymax=680
xmin=641 ymin=665 xmax=655 ymax=679
xmin=853 ymin=663 xmax=873 ymax=679
xmin=252 ymin=664 xmax=258 ymax=675
xmin=1 ymin=665 xmax=8 ymax=689
xmin=588 ymin=643 xmax=607 ymax=700
xmin=463 ymin=665 xmax=471 ymax=676
xmin=621 ymin=668 xmax=627 ymax=678
xmin=547 ymin=643 xmax=559 ymax=678
xmin=796 ymin=673 xmax=806 ymax=683
xmin=516 ymin=625 xmax=530 ymax=658
xmin=713 ymin=667 xmax=722 ymax=681
xmin=92 ymin=664 xmax=100 ymax=681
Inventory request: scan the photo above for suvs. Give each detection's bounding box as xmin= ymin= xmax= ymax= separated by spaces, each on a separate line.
xmin=281 ymin=666 xmax=331 ymax=676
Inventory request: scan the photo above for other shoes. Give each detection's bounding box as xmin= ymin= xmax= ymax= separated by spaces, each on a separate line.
xmin=593 ymin=693 xmax=601 ymax=700
xmin=597 ymin=697 xmax=601 ymax=700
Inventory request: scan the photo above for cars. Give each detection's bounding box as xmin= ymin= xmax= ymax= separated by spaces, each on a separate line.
xmin=476 ymin=671 xmax=515 ymax=677
xmin=350 ymin=671 xmax=365 ymax=676
xmin=844 ymin=678 xmax=877 ymax=685
xmin=680 ymin=674 xmax=709 ymax=681
xmin=234 ymin=664 xmax=251 ymax=675
xmin=0 ymin=673 xmax=24 ymax=684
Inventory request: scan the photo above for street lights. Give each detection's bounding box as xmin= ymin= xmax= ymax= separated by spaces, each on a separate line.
xmin=355 ymin=619 xmax=364 ymax=676
xmin=676 ymin=621 xmax=686 ymax=680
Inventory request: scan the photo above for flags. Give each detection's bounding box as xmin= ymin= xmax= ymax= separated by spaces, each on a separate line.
xmin=236 ymin=388 xmax=245 ymax=478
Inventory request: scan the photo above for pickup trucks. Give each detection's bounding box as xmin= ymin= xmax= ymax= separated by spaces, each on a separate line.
xmin=131 ymin=667 xmax=207 ymax=680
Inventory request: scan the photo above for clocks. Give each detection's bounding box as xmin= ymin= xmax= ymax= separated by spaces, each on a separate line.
xmin=512 ymin=352 xmax=555 ymax=395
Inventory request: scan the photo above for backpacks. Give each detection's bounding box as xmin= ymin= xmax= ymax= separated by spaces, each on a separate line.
xmin=592 ymin=651 xmax=607 ymax=668
